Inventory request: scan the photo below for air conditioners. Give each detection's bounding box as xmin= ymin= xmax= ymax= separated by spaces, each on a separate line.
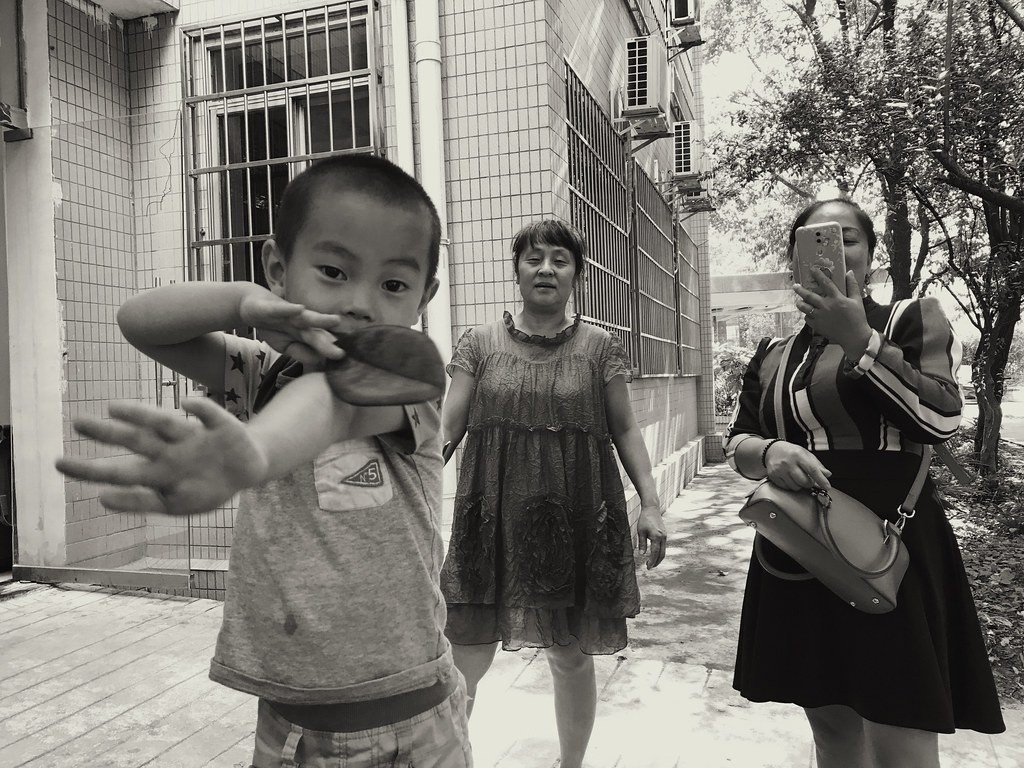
xmin=682 ymin=156 xmax=713 ymax=211
xmin=672 ymin=0 xmax=702 ymax=46
xmin=673 ymin=121 xmax=702 ymax=196
xmin=622 ymin=35 xmax=674 ymax=134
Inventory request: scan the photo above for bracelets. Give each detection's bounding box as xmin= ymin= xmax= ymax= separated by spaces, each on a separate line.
xmin=762 ymin=438 xmax=786 ymax=469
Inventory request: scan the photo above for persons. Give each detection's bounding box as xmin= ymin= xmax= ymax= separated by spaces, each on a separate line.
xmin=721 ymin=198 xmax=1006 ymax=768
xmin=438 ymin=219 xmax=668 ymax=768
xmin=55 ymin=154 xmax=474 ymax=768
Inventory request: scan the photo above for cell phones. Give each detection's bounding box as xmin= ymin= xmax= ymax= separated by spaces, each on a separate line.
xmin=796 ymin=221 xmax=847 ymax=297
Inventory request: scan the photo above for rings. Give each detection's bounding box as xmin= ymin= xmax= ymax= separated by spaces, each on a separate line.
xmin=808 ymin=306 xmax=816 ymax=318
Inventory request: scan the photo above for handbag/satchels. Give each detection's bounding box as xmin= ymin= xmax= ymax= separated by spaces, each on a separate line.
xmin=739 ymin=467 xmax=910 ymax=613
